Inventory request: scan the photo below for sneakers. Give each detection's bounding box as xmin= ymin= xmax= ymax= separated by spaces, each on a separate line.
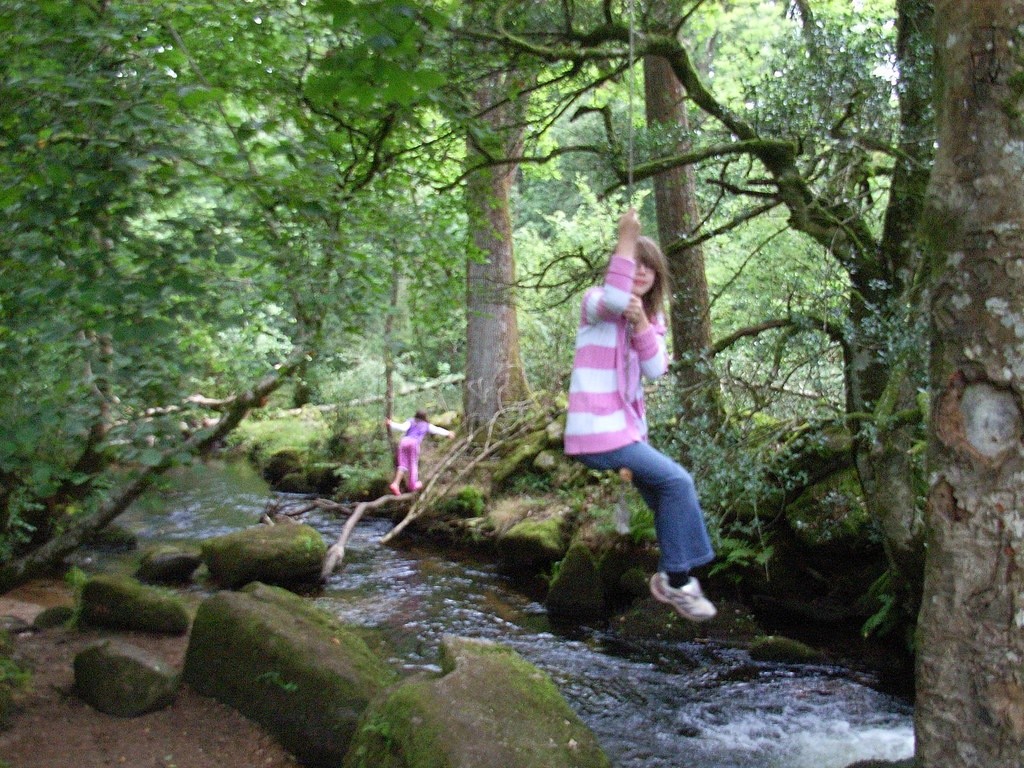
xmin=650 ymin=573 xmax=716 ymax=622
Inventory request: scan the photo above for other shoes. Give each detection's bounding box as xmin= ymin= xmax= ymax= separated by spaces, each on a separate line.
xmin=408 ymin=481 xmax=422 ymax=490
xmin=389 ymin=483 xmax=401 ymax=495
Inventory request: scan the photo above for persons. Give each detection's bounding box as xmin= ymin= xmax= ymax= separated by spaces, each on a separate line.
xmin=563 ymin=208 xmax=719 ymax=622
xmin=385 ymin=410 xmax=456 ymax=495
